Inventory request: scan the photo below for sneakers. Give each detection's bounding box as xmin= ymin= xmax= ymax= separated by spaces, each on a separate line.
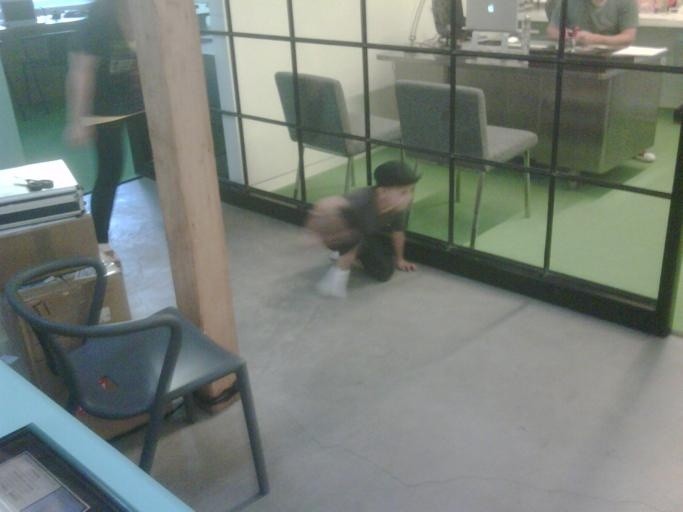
xmin=636 ymin=150 xmax=655 ymax=163
xmin=317 ymin=248 xmax=350 ymax=297
xmin=100 ymin=244 xmax=124 ymax=272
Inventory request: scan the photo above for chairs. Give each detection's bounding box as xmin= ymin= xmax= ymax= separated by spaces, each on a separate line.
xmin=274 ymin=71 xmax=403 ymax=204
xmin=16 ymin=28 xmax=76 ymax=121
xmin=395 ymin=79 xmax=540 ymax=247
xmin=2 ymin=257 xmax=268 ymax=496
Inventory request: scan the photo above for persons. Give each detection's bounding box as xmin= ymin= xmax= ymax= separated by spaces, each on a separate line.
xmin=546 ymin=1 xmax=657 ymax=165
xmin=60 ymin=0 xmax=158 ymax=272
xmin=303 ymin=159 xmax=422 ymax=296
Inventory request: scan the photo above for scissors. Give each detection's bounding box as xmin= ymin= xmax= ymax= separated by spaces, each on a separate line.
xmin=13 ymin=179 xmax=54 ymax=191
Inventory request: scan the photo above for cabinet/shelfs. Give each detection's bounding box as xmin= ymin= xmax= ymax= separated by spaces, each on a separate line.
xmin=1 ymin=213 xmax=101 ymax=388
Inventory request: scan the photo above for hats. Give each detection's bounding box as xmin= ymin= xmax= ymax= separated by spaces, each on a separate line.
xmin=374 ymin=160 xmax=420 ymax=187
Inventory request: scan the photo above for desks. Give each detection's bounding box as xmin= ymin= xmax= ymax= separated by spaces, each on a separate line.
xmin=0 ymin=12 xmax=84 ymax=102
xmin=1 ymin=357 xmax=195 ymax=512
xmin=377 ymin=32 xmax=668 ymax=188
xmin=519 ymin=8 xmax=683 ymax=126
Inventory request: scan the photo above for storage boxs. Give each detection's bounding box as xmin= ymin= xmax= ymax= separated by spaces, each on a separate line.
xmin=14 ymin=252 xmax=133 ymax=407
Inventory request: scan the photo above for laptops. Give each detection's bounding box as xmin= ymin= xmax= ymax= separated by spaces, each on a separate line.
xmin=467 ymin=0 xmax=540 ymax=33
